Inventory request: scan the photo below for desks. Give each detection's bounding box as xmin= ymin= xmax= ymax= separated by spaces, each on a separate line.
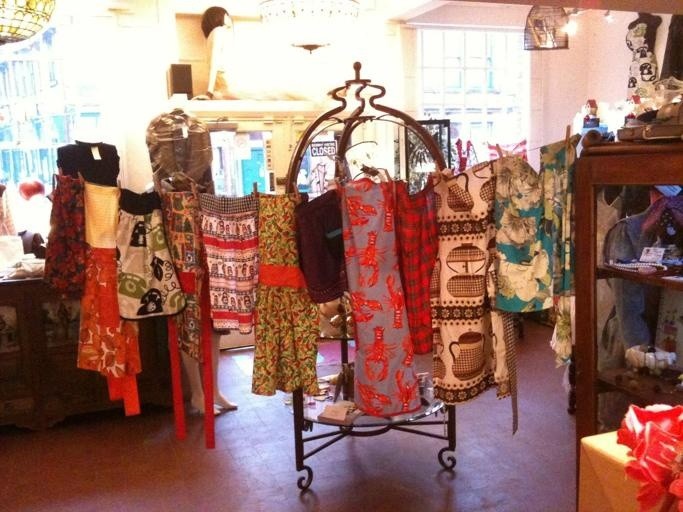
xmin=0 ymin=265 xmax=202 ymax=435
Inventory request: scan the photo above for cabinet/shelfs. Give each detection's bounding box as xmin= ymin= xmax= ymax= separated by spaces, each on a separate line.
xmin=569 ymin=142 xmax=682 ymax=507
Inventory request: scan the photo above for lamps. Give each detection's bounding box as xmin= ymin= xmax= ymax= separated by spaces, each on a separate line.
xmin=258 ymin=0 xmax=361 ymax=55
xmin=563 ymin=7 xmax=613 ymax=33
xmin=1 ymin=2 xmax=57 ymax=44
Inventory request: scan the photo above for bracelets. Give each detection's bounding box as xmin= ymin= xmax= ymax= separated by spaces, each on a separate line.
xmin=205 ymin=92 xmax=216 ymax=99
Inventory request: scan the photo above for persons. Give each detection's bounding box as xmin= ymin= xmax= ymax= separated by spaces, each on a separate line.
xmin=191 ymin=7 xmax=311 ymax=100
xmin=148 ymin=108 xmax=237 ymax=416
xmin=624 ymin=12 xmax=662 ymax=101
